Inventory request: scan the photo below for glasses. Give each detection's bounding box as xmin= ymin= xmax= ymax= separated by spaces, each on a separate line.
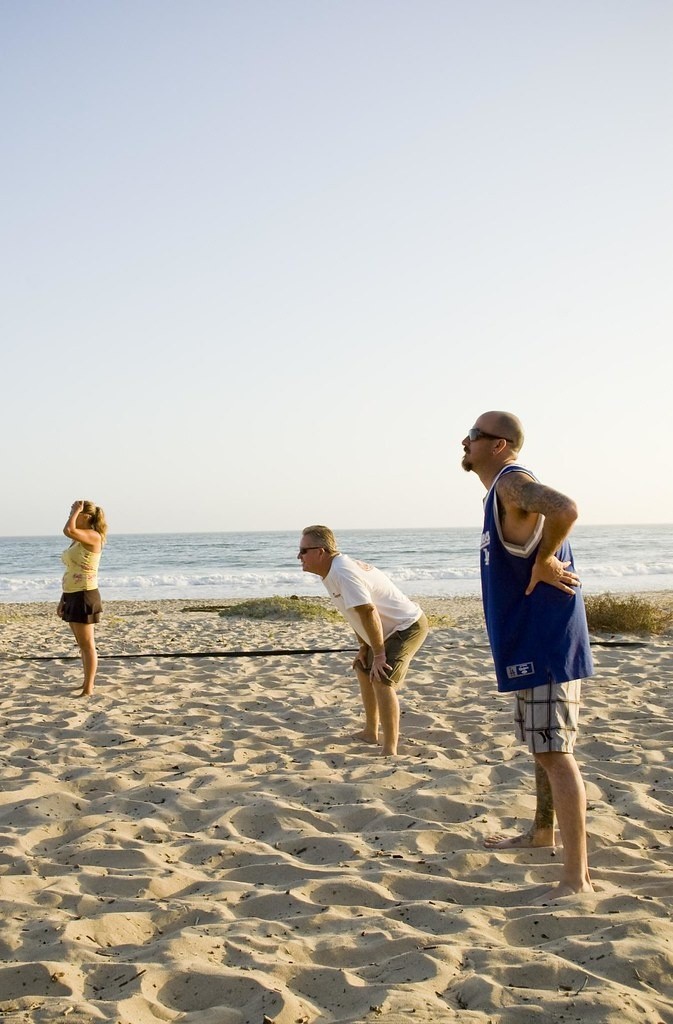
xmin=299 ymin=547 xmax=327 ymax=555
xmin=469 ymin=428 xmax=514 ymax=443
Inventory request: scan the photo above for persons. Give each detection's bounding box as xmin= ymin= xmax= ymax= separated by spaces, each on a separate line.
xmin=298 ymin=525 xmax=429 ymax=756
xmin=461 ymin=411 xmax=595 ymax=907
xmin=57 ymin=500 xmax=108 ymax=697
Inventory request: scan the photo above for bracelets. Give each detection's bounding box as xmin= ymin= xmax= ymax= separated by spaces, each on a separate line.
xmin=374 ymin=654 xmax=385 ymax=657
xmin=359 ymin=643 xmax=365 ymax=647
